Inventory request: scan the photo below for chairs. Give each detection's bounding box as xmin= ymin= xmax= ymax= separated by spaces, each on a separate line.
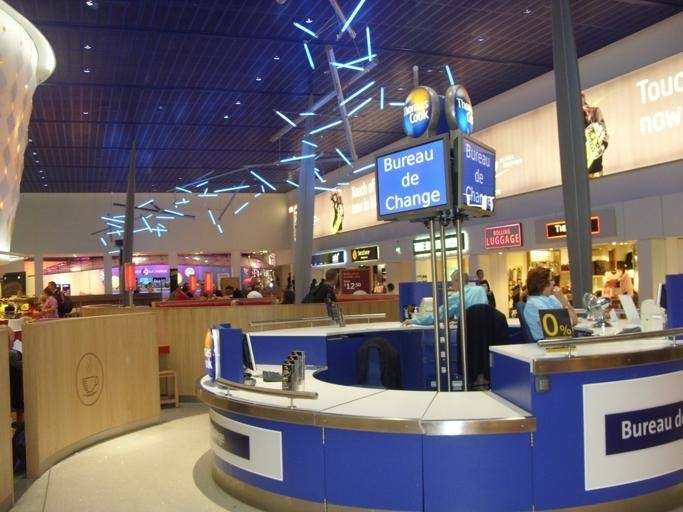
xmin=354 ymin=337 xmax=403 ymax=389
xmin=157 ymin=345 xmax=179 ymax=407
xmin=457 ymin=305 xmax=510 ymax=391
xmin=515 ymin=300 xmax=539 ymax=342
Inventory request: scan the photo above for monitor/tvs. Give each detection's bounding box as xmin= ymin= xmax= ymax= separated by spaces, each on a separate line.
xmin=451 ymin=130 xmax=497 ymax=216
xmin=376 ymin=132 xmax=454 ymax=221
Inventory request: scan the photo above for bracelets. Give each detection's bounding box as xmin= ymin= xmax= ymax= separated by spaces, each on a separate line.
xmin=564 ymin=300 xmax=570 ymax=310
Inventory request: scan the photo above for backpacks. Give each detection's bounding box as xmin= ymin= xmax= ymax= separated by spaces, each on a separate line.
xmin=305 ymin=285 xmax=327 ymax=303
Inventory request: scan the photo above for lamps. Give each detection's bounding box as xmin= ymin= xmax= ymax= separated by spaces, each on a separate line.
xmin=188 ymin=271 xmax=213 ymax=301
xmin=123 ymin=262 xmax=136 ymax=308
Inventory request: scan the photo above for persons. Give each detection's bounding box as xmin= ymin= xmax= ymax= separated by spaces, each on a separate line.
xmin=602 ymin=259 xmax=638 ymax=299
xmin=521 ymin=265 xmax=579 ymax=342
xmin=329 ymin=195 xmax=344 ymax=236
xmin=44 ymin=309 xmax=47 ymax=314
xmin=512 ymin=278 xmax=527 ymax=321
xmin=582 ymin=92 xmax=609 ymax=179
xmin=329 ymin=190 xmax=342 ymax=233
xmin=0 ymin=324 xmax=23 ymax=412
xmin=10 ymin=288 xmax=25 ymax=297
xmin=46 ymin=280 xmax=64 ymax=318
xmin=133 ymin=282 xmax=153 ymax=294
xmin=303 ymin=268 xmax=397 ymax=303
xmin=179 ymin=272 xmax=294 ymax=305
xmin=33 ymin=287 xmax=59 ymax=318
xmin=475 ymin=269 xmax=489 ymax=295
xmin=400 ymin=269 xmax=490 ymax=326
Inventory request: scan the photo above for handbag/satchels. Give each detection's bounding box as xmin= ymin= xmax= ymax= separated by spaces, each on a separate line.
xmin=62 ymin=297 xmax=73 ymax=313
